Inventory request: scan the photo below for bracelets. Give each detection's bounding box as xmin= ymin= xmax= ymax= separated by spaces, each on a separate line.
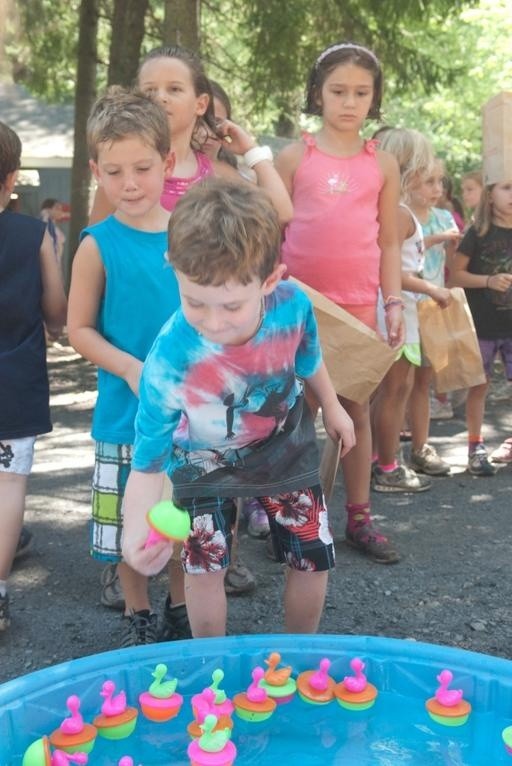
xmin=383 ymin=296 xmax=405 ymax=312
xmin=381 ymin=294 xmax=405 ymax=311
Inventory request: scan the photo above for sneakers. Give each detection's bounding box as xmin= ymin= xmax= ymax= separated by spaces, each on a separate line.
xmin=161 ymin=604 xmax=193 ymax=641
xmin=344 ymin=525 xmax=400 ymax=564
xmin=467 ymin=445 xmax=497 ymax=476
xmin=243 ymin=496 xmax=272 ymax=539
xmin=99 ymin=564 xmax=126 ymax=611
xmin=404 ymin=442 xmax=451 ymax=477
xmin=489 ymin=435 xmax=512 ymax=465
xmin=372 ymin=463 xmax=432 ymax=493
xmin=119 ymin=609 xmax=157 ymax=649
xmin=224 ymin=560 xmax=256 ymax=593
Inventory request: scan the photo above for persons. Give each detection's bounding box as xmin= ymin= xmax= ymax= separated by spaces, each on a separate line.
xmin=19 ymin=733 xmax=86 ymax=766
xmin=66 ymin=85 xmax=196 ymax=647
xmin=38 ymin=198 xmax=67 ymax=346
xmin=85 ymin=45 xmax=297 ymax=609
xmin=264 ymin=42 xmax=406 ymax=565
xmin=120 ymin=178 xmax=359 ymax=639
xmin=0 ymin=122 xmax=70 ymax=631
xmin=429 ymin=170 xmax=464 ymax=287
xmin=460 ymin=172 xmax=493 ymax=217
xmin=466 ymin=91 xmax=512 ymax=466
xmin=187 ymin=80 xmax=269 ymax=538
xmin=406 ymin=155 xmax=462 ymax=476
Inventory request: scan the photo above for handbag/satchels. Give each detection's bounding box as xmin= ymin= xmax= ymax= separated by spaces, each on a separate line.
xmin=480 ymin=92 xmax=512 ymax=186
xmin=282 ymin=278 xmax=401 ymax=406
xmin=417 ymin=290 xmax=488 ymax=399
